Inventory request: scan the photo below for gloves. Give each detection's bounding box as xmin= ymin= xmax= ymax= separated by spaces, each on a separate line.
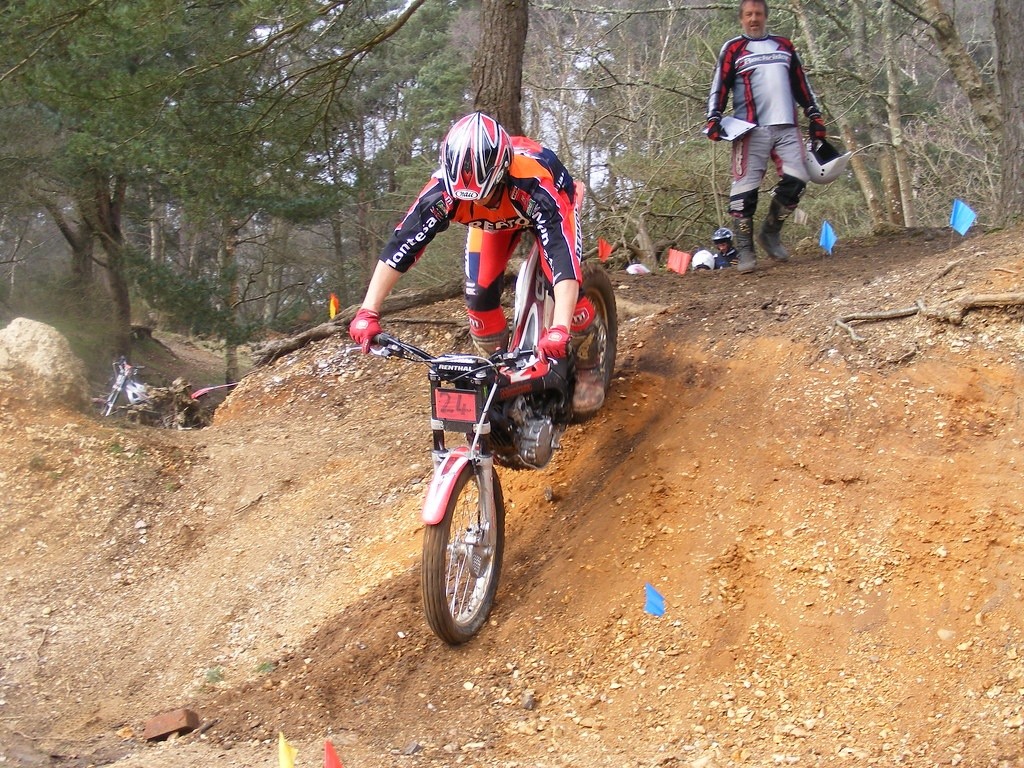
xmin=347 ymin=308 xmax=384 ymax=355
xmin=536 ymin=324 xmax=572 ymax=365
xmin=706 ymin=116 xmax=728 ymax=142
xmin=806 ymin=112 xmax=828 ymax=141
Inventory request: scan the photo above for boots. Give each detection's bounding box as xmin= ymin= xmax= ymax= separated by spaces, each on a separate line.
xmin=732 ymin=215 xmax=757 ymax=273
xmin=569 ymin=312 xmax=607 ymax=413
xmin=470 ymin=320 xmax=509 ymax=358
xmin=756 ymin=198 xmax=792 ymax=263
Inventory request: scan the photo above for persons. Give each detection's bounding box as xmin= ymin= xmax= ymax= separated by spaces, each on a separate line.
xmin=707 ymin=0 xmax=827 ymax=272
xmin=692 ymin=250 xmax=715 ymax=271
xmin=711 ymin=228 xmax=739 ymax=269
xmin=349 ymin=110 xmax=606 ymax=412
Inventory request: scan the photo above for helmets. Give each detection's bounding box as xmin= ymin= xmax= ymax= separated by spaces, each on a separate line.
xmin=710 ymin=227 xmax=734 ymax=241
xmin=804 ymin=137 xmax=854 ymax=184
xmin=440 ymin=111 xmax=515 ymax=201
xmin=692 ymin=250 xmax=715 ymax=270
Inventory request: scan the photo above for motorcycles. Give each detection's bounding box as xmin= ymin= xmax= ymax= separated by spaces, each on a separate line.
xmin=94 ymin=352 xmax=171 ymax=418
xmin=343 ymin=250 xmax=618 ymax=647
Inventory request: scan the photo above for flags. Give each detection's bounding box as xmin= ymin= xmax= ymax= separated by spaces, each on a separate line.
xmin=330 ymin=294 xmax=339 ymax=318
xmin=644 ymin=582 xmax=665 ymax=615
xmin=599 ymin=238 xmax=614 ymax=263
xmin=795 ymin=209 xmax=808 ymax=225
xmin=820 ymin=221 xmax=837 ymax=255
xmin=326 ymin=743 xmax=342 ymax=768
xmin=950 ymin=199 xmax=976 ymax=236
xmin=668 ymin=249 xmax=692 ymax=275
xmin=279 ymin=733 xmax=298 ymax=768
